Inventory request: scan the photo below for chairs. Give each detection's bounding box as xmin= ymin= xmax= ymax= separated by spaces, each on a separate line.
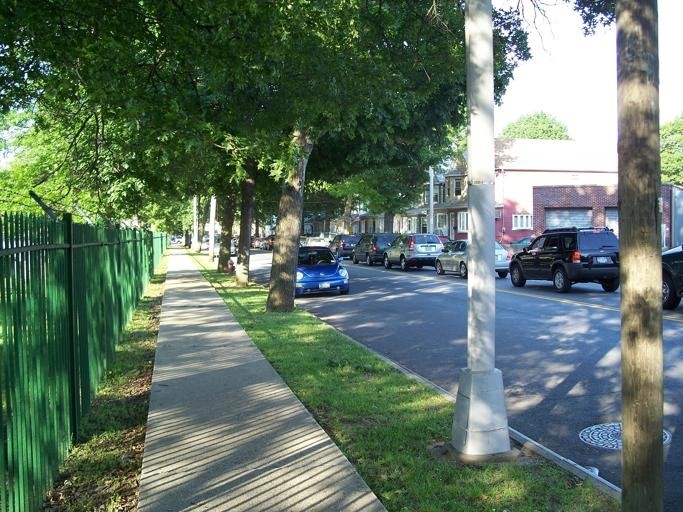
xmin=309 ymin=254 xmax=331 ymax=265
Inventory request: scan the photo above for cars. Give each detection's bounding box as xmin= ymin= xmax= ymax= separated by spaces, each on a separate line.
xmin=328 ymin=234 xmax=360 ymax=259
xmin=293 ymin=246 xmax=350 ymax=298
xmin=170 ymin=237 xmax=182 ymax=245
xmin=351 ymin=232 xmax=401 ymax=266
xmin=212 ymin=232 xmax=338 ymax=251
xmin=510 ymin=226 xmax=683 ymax=311
xmin=382 ymin=233 xmax=445 ymax=272
xmin=434 ymin=239 xmax=512 ymax=279
